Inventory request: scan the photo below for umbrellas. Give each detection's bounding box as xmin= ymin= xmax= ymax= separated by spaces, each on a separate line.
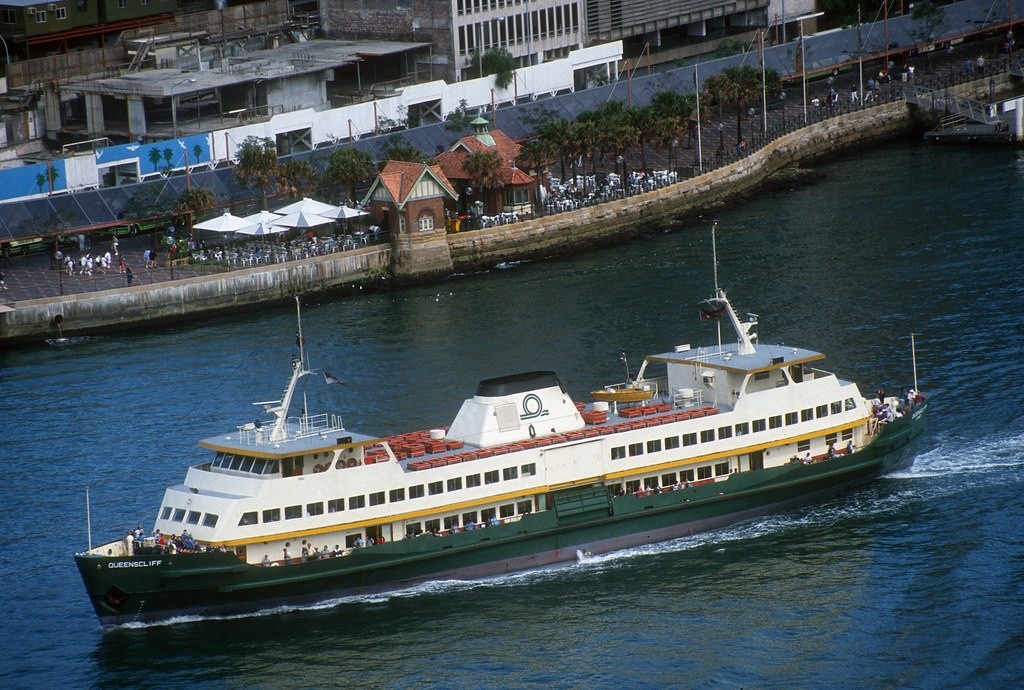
xmin=192 ymin=197 xmax=369 ymax=235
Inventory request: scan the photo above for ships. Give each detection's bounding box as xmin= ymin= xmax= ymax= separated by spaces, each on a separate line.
xmin=68 ymin=222 xmax=943 ymax=629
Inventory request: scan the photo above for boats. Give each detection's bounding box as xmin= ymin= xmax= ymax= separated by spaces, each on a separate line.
xmin=590 ymin=389 xmax=653 ymax=402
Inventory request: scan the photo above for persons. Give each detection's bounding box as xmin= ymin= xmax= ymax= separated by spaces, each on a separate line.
xmin=873 ymin=387 xmax=923 ymax=422
xmin=619 ymin=477 xmax=692 ymax=497
xmin=369 ymin=223 xmax=381 ymax=241
xmin=603 ymin=177 xmax=613 ymax=189
xmin=965 ymin=54 xmax=984 ymax=77
xmin=206 ymin=544 xmax=226 ymax=552
xmin=144 ymin=224 xmax=194 ymax=269
xmin=432 ymin=514 xmax=499 ymax=537
xmin=627 ymin=171 xmax=648 ymax=186
xmin=65 ymin=233 xmax=127 ymax=276
xmin=130 ymin=526 xmax=200 ymax=554
xmin=302 ymin=536 xmax=386 ymax=561
xmin=792 ymin=441 xmax=854 ymax=461
xmin=263 ymin=555 xmax=270 ymax=566
xmin=739 ymin=141 xmax=745 ymax=160
xmin=283 ymin=548 xmax=291 ymax=565
xmin=864 ymin=64 xmax=916 ymax=101
xmin=811 ymin=84 xmax=858 ymax=112
xmin=551 ymin=183 xmax=578 ymax=198
xmin=129 ymin=224 xmax=137 ymax=240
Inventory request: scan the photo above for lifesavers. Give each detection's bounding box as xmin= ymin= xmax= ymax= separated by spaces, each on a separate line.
xmin=324 ymin=462 xmax=332 ymax=472
xmin=313 ymin=464 xmax=323 ymax=473
xmin=347 ymin=458 xmax=357 ymax=468
xmin=285 ymin=470 xmax=297 ymax=477
xmin=335 ymin=459 xmax=346 ymax=469
